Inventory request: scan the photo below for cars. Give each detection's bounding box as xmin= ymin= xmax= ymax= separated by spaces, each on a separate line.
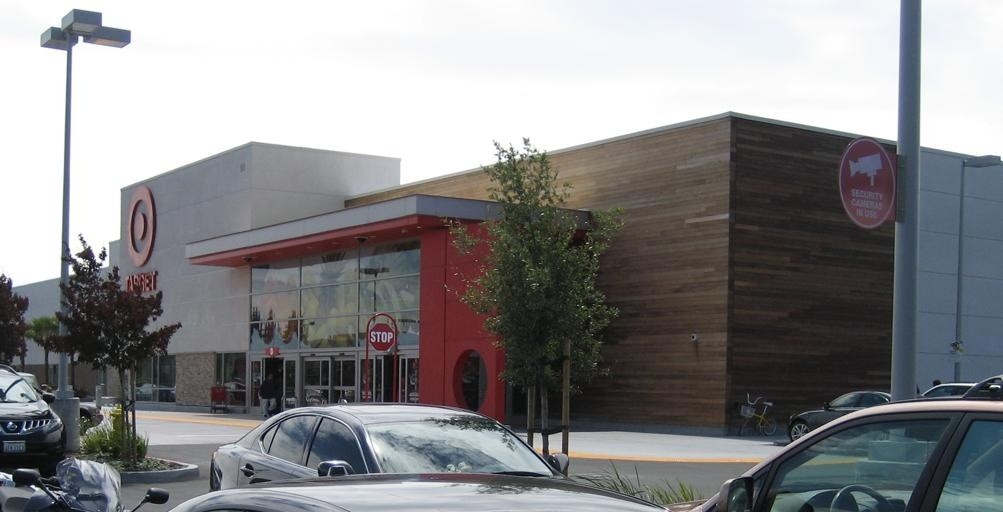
xmin=208 ymin=403 xmax=578 ymax=484
xmin=172 ymin=471 xmax=679 ymax=512
xmin=223 ymin=378 xmax=327 ymax=410
xmin=33 ymin=386 xmax=104 ymax=434
xmin=135 ymin=384 xmax=170 ymax=402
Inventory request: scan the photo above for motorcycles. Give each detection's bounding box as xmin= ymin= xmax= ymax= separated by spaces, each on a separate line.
xmin=1 ymin=456 xmax=171 ymax=512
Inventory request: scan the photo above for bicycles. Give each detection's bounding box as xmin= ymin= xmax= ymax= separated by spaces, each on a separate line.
xmin=738 ymin=390 xmax=778 ymax=436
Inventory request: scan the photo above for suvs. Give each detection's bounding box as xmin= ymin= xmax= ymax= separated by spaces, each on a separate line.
xmin=668 ymin=370 xmax=1001 ymax=510
xmin=0 ymin=365 xmax=64 ymax=467
xmin=788 ymin=382 xmax=1001 ymax=462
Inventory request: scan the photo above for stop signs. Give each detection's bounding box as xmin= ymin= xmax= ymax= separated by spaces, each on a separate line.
xmin=367 ymin=323 xmax=396 ymax=354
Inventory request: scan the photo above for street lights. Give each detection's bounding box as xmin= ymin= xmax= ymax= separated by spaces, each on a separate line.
xmin=37 ymin=7 xmax=132 ymax=450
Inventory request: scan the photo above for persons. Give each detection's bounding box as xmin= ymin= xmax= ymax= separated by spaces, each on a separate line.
xmin=259 ymin=373 xmax=277 ymax=417
